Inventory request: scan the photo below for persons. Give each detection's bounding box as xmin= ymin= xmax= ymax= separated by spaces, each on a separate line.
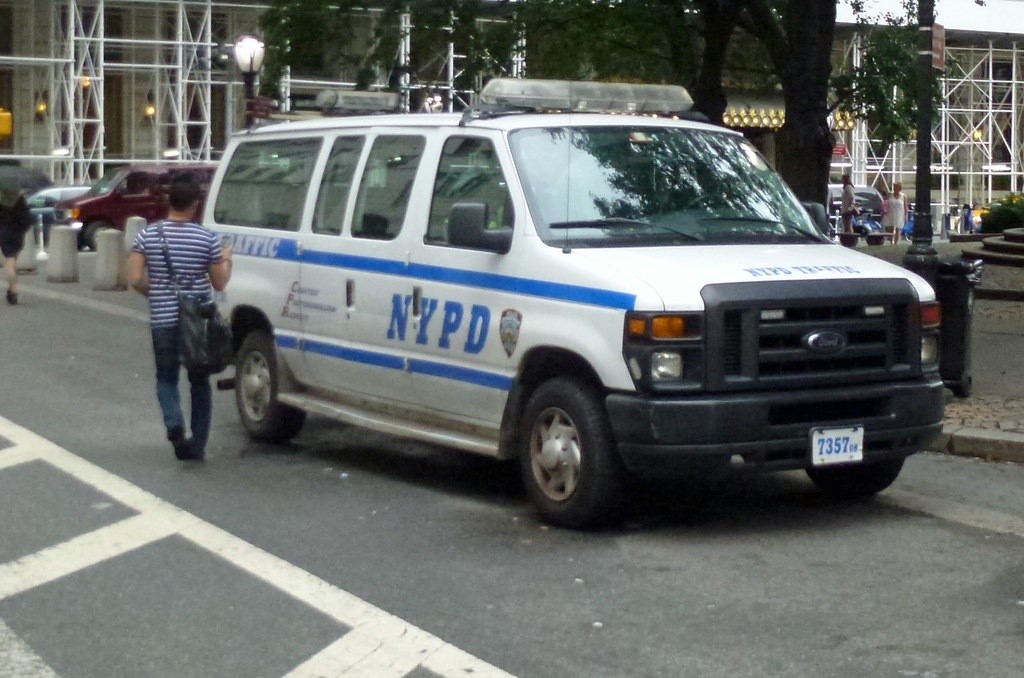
xmin=0 ymin=188 xmax=33 ymax=304
xmin=841 ymin=174 xmax=856 ymax=233
xmin=130 ymin=170 xmax=232 ymax=459
xmin=879 ymin=182 xmax=907 ymax=245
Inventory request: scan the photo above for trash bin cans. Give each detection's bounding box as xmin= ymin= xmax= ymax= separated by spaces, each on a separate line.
xmin=937 ymin=257 xmax=984 ymax=398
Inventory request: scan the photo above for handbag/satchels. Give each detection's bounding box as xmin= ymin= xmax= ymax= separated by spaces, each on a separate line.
xmin=177 ymin=294 xmax=233 ymax=375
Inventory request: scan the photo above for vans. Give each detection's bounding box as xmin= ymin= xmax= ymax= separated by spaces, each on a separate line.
xmin=829 ymin=182 xmax=883 ymax=229
xmin=53 ymin=163 xmax=218 ymax=252
xmin=0 ymin=152 xmax=70 ymax=199
xmin=198 ymin=75 xmax=952 ymax=533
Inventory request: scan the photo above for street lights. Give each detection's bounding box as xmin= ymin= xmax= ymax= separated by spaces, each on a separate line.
xmin=231 ymin=37 xmax=269 ymax=127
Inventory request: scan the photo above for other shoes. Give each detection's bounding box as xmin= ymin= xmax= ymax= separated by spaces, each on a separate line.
xmin=6 ymin=289 xmax=17 ymax=304
xmin=171 ymin=430 xmax=191 ymax=461
xmin=187 ymin=438 xmax=204 ymax=459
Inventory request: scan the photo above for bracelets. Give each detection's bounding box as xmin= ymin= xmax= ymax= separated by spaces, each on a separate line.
xmin=224 ymin=259 xmax=232 ymax=262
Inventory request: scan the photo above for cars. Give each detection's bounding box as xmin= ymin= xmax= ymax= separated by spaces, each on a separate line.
xmin=25 ymin=185 xmax=110 ymax=246
